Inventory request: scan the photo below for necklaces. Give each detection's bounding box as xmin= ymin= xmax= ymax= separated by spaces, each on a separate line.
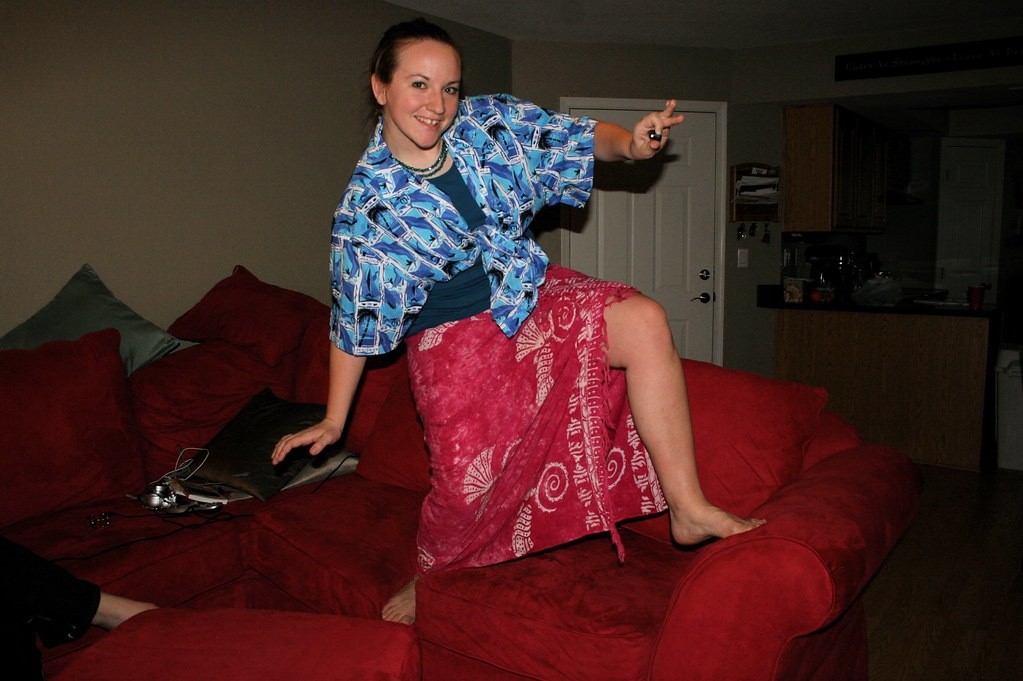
xmin=395 ymin=140 xmax=449 ymax=178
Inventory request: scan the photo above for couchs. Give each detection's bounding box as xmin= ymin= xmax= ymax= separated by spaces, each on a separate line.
xmin=0 ymin=333 xmax=916 ymax=681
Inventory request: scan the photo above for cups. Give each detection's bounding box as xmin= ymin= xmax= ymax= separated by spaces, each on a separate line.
xmin=968 ymin=283 xmax=985 ymax=310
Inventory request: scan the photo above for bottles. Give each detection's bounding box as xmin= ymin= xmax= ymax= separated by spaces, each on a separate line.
xmin=780 ymin=246 xmax=805 ymax=283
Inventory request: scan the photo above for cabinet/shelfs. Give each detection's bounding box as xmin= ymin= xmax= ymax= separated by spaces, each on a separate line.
xmin=780 ymin=103 xmax=925 ymax=234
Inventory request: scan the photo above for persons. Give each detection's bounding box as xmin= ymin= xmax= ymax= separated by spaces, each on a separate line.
xmin=0 ymin=539 xmax=159 ymax=681
xmin=271 ymin=18 xmax=771 ymax=624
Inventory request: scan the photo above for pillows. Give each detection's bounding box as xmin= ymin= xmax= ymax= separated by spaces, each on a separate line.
xmin=1 ymin=260 xmax=828 ymax=545
